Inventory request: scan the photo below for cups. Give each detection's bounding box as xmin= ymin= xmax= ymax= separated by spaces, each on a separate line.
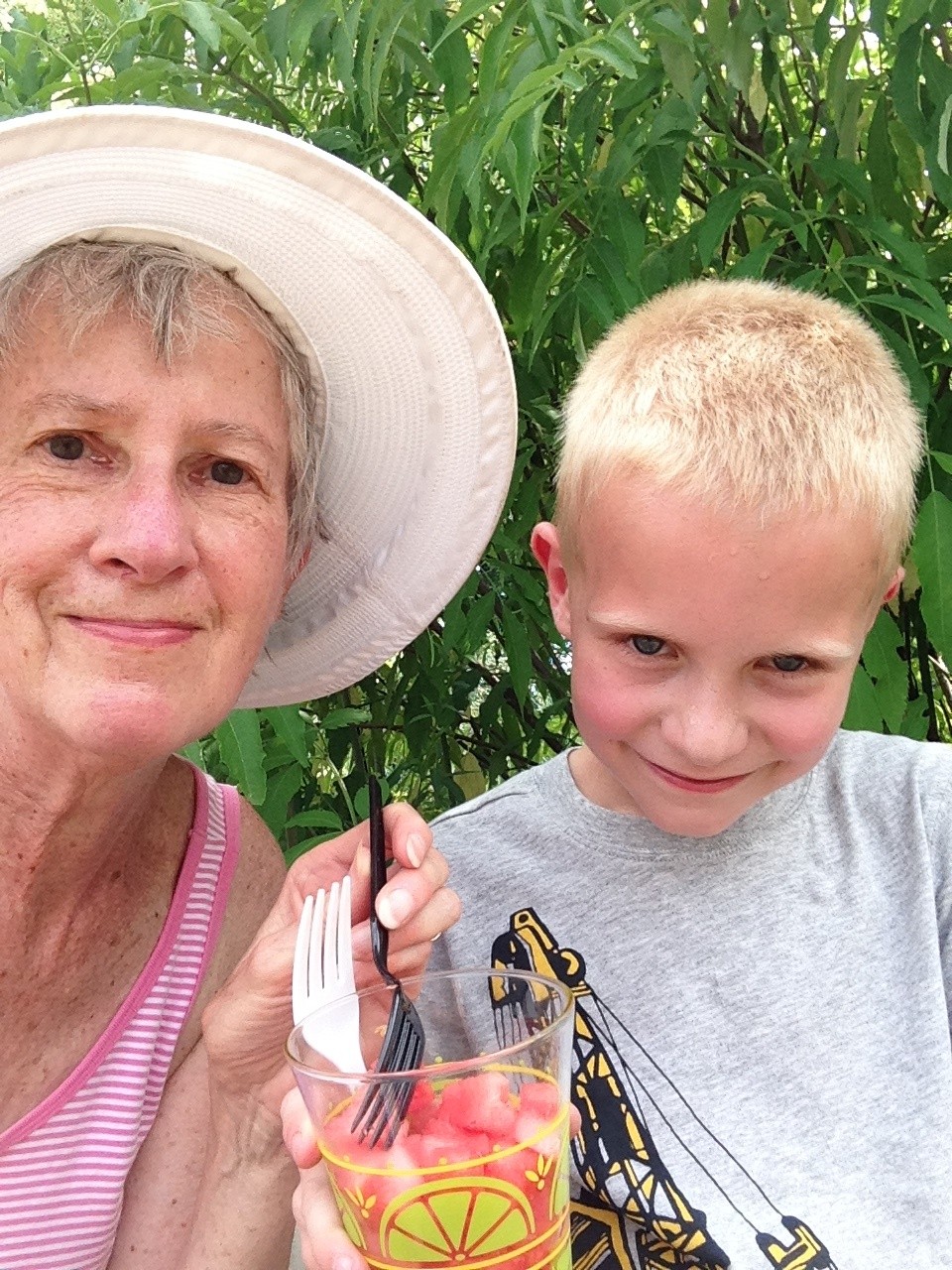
xmin=283 ymin=968 xmax=577 ymax=1270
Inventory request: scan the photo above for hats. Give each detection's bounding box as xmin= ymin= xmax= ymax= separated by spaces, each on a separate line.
xmin=0 ymin=105 xmax=519 ymax=709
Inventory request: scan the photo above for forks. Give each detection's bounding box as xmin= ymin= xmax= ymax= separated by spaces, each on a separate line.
xmin=349 ymin=773 xmax=426 ymax=1151
xmin=291 ymin=873 xmax=367 ymax=1092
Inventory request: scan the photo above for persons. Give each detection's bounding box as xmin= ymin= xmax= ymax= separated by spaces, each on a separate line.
xmin=286 ymin=280 xmax=952 ymax=1270
xmin=1 ymin=104 xmax=466 ymax=1270
